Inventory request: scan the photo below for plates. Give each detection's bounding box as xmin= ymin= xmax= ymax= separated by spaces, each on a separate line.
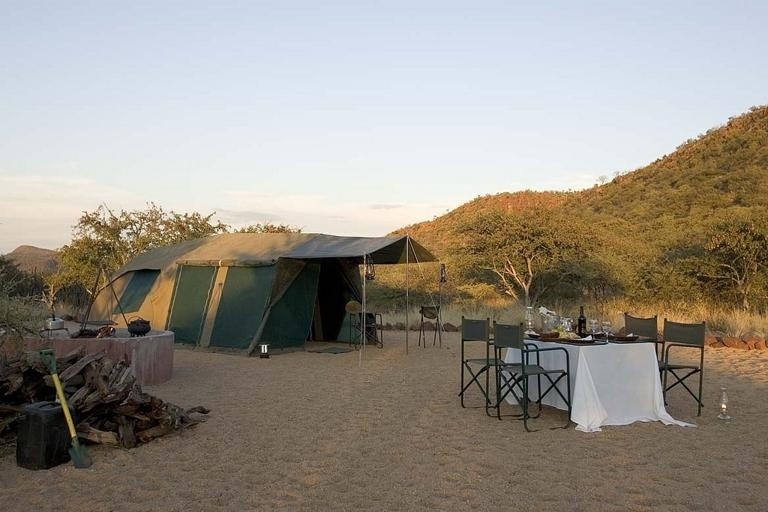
xmin=526 ymin=305 xmax=639 ymax=343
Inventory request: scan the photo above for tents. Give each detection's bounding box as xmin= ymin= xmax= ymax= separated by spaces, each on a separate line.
xmin=84 ymin=231 xmax=379 ymax=358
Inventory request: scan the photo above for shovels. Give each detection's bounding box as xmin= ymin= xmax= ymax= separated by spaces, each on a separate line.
xmin=40 ymin=349 xmax=92 ymax=468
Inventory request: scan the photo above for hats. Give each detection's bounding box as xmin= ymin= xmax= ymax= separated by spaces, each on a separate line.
xmin=345 ymin=301 xmax=361 ymax=314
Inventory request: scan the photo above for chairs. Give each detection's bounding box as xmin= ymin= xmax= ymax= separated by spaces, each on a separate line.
xmin=459 ymin=310 xmax=706 ymax=433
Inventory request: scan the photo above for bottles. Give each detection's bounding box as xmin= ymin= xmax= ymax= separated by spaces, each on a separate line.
xmin=719 ymin=387 xmax=729 ymax=418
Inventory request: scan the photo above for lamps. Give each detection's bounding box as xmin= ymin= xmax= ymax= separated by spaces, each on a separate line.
xmin=716 ymin=387 xmax=734 ymax=421
xmin=365 ymin=255 xmax=376 ymax=280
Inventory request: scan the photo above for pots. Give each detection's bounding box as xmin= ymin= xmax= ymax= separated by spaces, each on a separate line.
xmin=127 ymin=319 xmax=151 ymax=335
xmin=44 ymin=314 xmax=64 ymax=330
